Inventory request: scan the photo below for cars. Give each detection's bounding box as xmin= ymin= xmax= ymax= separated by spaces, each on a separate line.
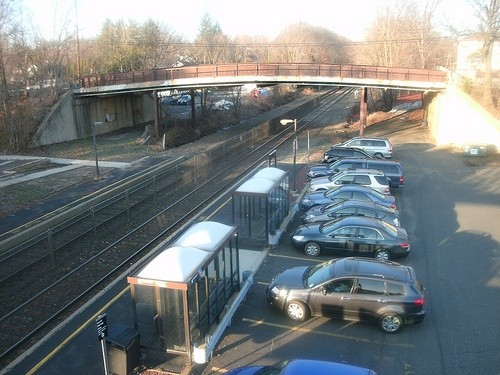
xmin=312 ymin=169 xmax=399 ymax=198
xmin=303 ymin=184 xmax=397 ymax=212
xmin=264 ymin=256 xmax=427 ymax=334
xmin=322 ymin=147 xmax=377 ymax=164
xmin=171 ymin=94 xmax=192 ymax=106
xmin=300 ymin=200 xmax=401 ymax=230
xmin=290 ymin=216 xmax=411 ymax=262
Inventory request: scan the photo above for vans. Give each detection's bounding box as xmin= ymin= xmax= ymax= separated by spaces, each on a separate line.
xmin=307 ymin=157 xmax=404 ymax=190
xmin=330 ymin=137 xmax=393 ymax=163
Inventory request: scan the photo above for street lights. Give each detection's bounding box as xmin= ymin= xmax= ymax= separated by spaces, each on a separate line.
xmin=280 ymin=117 xmax=297 ymax=194
xmin=93 ymin=121 xmax=103 ymax=177
